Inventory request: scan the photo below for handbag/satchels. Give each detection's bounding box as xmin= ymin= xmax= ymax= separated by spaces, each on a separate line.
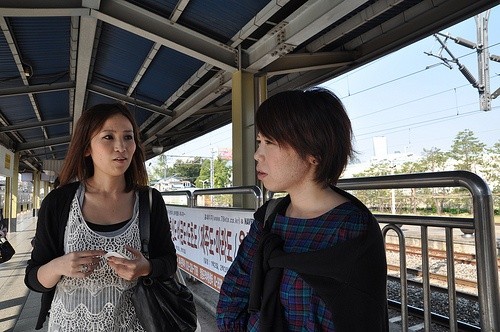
xmin=130 ymin=186 xmax=197 ymax=332
xmin=0 ymin=235 xmax=15 ymax=264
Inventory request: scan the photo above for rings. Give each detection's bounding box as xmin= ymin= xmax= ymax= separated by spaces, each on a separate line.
xmin=80 ymin=264 xmax=89 ymax=273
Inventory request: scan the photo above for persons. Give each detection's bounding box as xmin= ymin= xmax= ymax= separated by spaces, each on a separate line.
xmin=24 ymin=103 xmax=177 ymax=332
xmin=216 ymin=87 xmax=390 ymax=332
